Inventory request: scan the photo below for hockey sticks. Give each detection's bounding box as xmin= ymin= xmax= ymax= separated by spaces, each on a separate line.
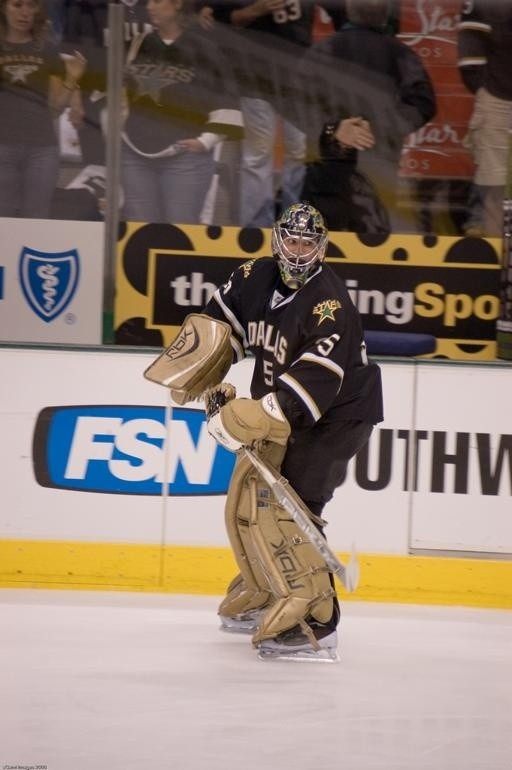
xmin=245 ymin=448 xmax=359 ymax=592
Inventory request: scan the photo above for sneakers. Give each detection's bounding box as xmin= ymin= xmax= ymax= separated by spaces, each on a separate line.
xmin=260 ymin=594 xmax=342 ymax=653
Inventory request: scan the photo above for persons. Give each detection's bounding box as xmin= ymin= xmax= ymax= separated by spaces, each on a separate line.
xmin=0 ymin=1 xmax=510 ymax=242
xmin=145 ymin=198 xmax=383 ymax=657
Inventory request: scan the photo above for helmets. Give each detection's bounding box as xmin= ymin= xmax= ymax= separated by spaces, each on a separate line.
xmin=271 ymin=202 xmax=330 ymax=290
